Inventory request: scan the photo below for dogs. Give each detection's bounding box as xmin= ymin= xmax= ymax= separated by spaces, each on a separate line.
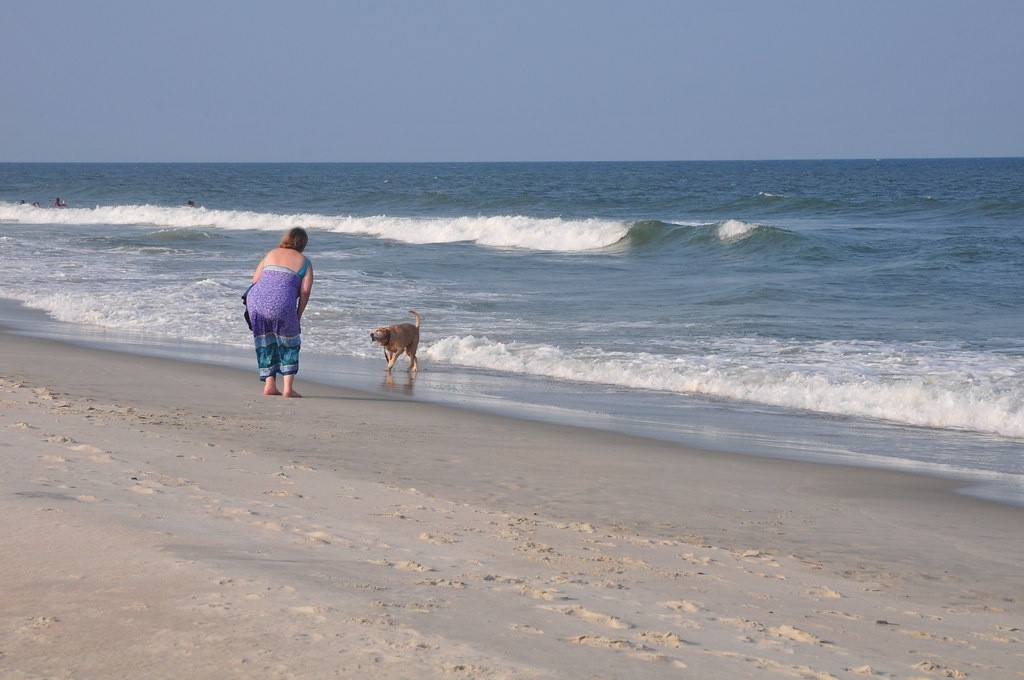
xmin=370 ymin=310 xmax=420 ymax=372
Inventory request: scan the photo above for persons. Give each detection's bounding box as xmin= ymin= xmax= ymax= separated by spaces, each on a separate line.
xmin=241 ymin=226 xmax=313 ymax=398
xmin=188 ymin=201 xmax=194 ymax=207
xmin=54 ymin=198 xmax=60 ymax=208
xmin=34 ymin=202 xmax=40 ymax=208
xmin=21 ymin=200 xmax=25 ymax=204
